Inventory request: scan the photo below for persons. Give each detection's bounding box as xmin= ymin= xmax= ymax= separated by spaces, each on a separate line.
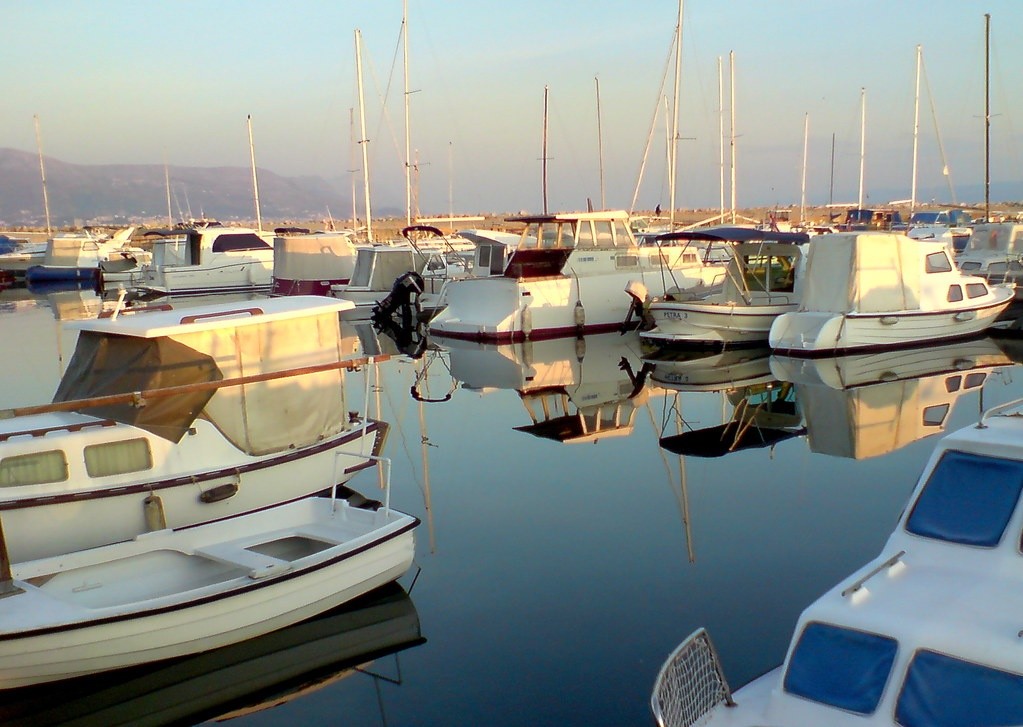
xmin=655 ymin=204 xmax=662 ymax=220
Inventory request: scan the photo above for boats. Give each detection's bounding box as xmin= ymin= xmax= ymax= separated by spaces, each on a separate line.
xmin=769 ymin=224 xmax=1016 ymax=360
xmin=0 ymin=284 xmax=390 ymax=558
xmin=423 ymin=329 xmax=685 ymax=445
xmin=0 ymin=579 xmax=428 ymax=727
xmin=0 ymin=441 xmax=421 ymax=692
xmin=638 ymin=225 xmax=838 ymax=354
xmin=649 ymin=399 xmax=1023 ymax=727
xmin=640 ymin=342 xmax=809 ymax=452
xmin=768 ymin=332 xmax=1023 ymax=461
xmin=426 ymin=210 xmax=731 ymax=344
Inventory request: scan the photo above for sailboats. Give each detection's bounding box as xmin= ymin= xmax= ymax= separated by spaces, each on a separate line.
xmin=1 ymin=0 xmax=1023 ymax=306
